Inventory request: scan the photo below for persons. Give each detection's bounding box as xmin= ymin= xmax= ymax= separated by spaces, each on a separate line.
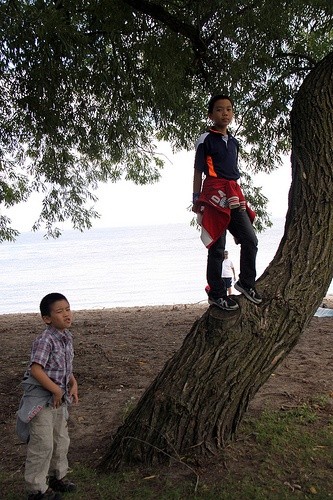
xmin=192 ymin=94 xmax=262 ymax=311
xmin=14 ymin=293 xmax=76 ymax=500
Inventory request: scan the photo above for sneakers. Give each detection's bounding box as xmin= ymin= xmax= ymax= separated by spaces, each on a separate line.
xmin=208 ymin=296 xmax=239 ymax=310
xmin=28 ymin=488 xmax=65 ymax=500
xmin=234 ymin=278 xmax=262 ymax=304
xmin=49 ymin=476 xmax=76 ymax=493
